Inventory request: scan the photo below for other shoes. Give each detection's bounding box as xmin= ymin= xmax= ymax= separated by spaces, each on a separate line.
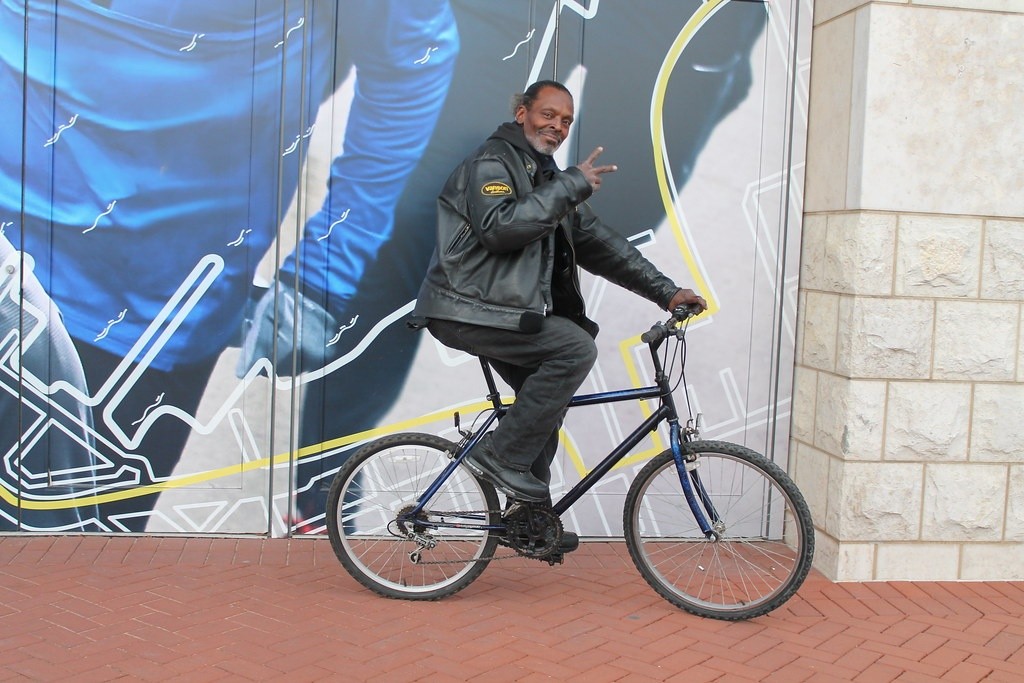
xmin=280 ymin=516 xmax=329 ymax=538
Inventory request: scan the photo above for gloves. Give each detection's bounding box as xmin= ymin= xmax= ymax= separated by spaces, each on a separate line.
xmin=236 ymin=280 xmax=338 ymax=380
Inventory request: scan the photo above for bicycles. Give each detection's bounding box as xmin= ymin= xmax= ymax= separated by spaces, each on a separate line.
xmin=325 ymin=304 xmax=816 ymax=622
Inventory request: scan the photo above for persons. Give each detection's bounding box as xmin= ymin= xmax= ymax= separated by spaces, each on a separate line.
xmin=294 ymin=0 xmax=769 ymax=533
xmin=2 ymin=0 xmax=460 ymax=533
xmin=405 ymin=79 xmax=706 ymax=551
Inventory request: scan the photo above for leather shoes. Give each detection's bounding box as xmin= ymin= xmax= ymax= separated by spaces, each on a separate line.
xmin=496 ymin=521 xmax=579 ymax=553
xmin=461 ymin=431 xmax=550 ymax=503
